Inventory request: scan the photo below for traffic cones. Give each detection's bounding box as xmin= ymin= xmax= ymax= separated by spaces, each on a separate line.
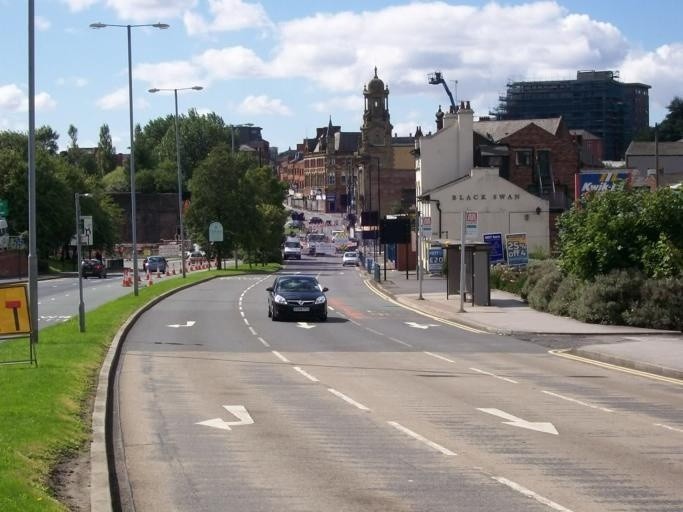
xmin=117 ymin=259 xmax=211 ymax=288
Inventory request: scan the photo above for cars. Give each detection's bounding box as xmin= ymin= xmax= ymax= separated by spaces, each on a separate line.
xmin=265 ymin=275 xmax=330 ymax=325
xmin=341 ymin=251 xmax=361 ymax=268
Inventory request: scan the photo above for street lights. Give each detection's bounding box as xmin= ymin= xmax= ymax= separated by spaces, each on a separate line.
xmin=229 ymin=121 xmax=258 ymax=158
xmin=74 ymin=191 xmax=96 ymax=334
xmin=351 ymin=154 xmax=387 ymax=283
xmin=85 ymin=19 xmax=171 ymax=299
xmin=146 ymin=84 xmax=206 ymax=280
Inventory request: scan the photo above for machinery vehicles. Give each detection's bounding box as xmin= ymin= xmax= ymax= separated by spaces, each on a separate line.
xmin=282 ymin=210 xmax=344 ymax=263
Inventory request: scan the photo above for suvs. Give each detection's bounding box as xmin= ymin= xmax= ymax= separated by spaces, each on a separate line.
xmin=186 ymin=252 xmax=203 ymax=266
xmin=78 ymin=258 xmax=107 ymax=280
xmin=145 ymin=256 xmax=169 ymax=274
